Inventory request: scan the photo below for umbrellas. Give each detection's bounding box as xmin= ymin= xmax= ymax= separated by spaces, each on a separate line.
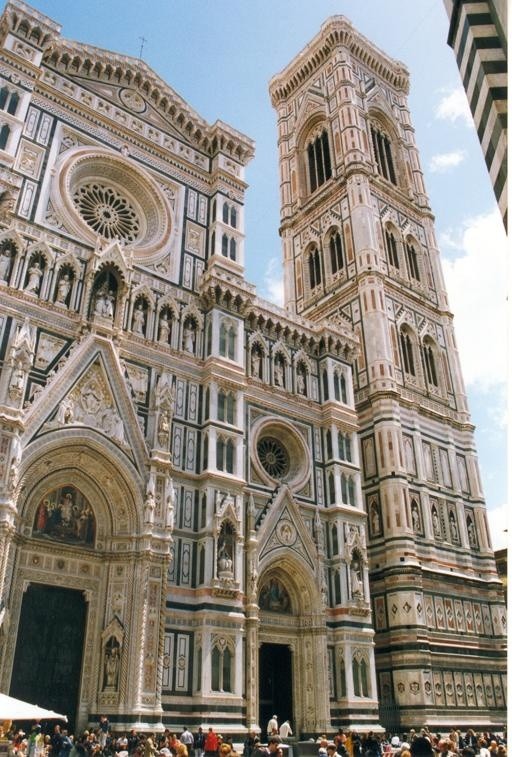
xmin=0 ymin=693 xmax=69 ymax=723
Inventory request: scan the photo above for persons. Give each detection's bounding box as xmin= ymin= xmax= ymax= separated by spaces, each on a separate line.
xmin=184 ymin=324 xmax=195 ymax=353
xmin=26 ymin=262 xmax=42 ymax=293
xmin=251 ymin=350 xmax=261 ymax=378
xmin=297 ymin=371 xmax=305 ymax=393
xmin=318 ymin=726 xmax=508 ymax=757
xmin=95 ymin=287 xmax=115 ymax=316
xmin=56 ymin=274 xmax=71 ymax=303
xmin=0 ymin=714 xmax=292 ymax=757
xmin=274 ymin=361 xmax=284 ymax=387
xmin=0 ymin=249 xmax=12 ymax=282
xmin=132 ymin=305 xmax=145 ymax=333
xmin=160 ymin=314 xmax=171 ymax=343
